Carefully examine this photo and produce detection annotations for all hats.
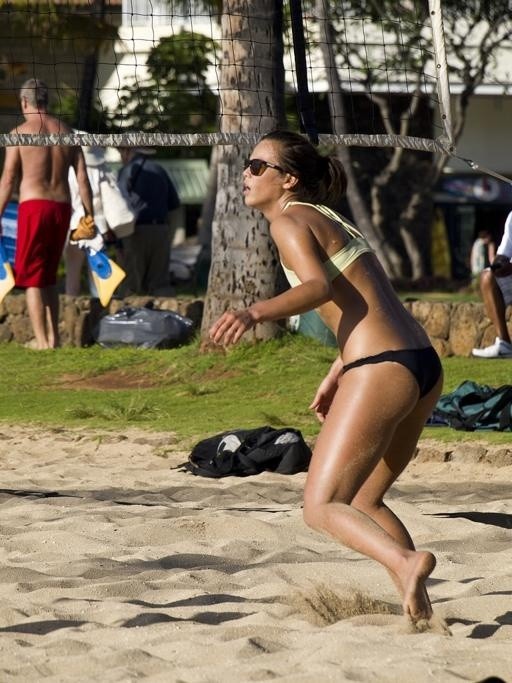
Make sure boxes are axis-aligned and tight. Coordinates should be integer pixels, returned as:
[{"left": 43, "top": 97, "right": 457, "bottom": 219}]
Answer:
[{"left": 72, "top": 130, "right": 105, "bottom": 167}]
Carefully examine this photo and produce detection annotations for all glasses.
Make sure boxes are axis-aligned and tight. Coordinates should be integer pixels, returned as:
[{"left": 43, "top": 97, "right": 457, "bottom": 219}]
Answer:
[{"left": 243, "top": 156, "right": 286, "bottom": 177}]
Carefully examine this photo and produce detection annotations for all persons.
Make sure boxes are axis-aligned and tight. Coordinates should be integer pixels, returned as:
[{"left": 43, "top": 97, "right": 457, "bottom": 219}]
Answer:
[
  {"left": 467, "top": 229, "right": 495, "bottom": 277},
  {"left": 0, "top": 76, "right": 98, "bottom": 352},
  {"left": 115, "top": 129, "right": 183, "bottom": 298},
  {"left": 208, "top": 129, "right": 447, "bottom": 626},
  {"left": 64, "top": 129, "right": 123, "bottom": 295},
  {"left": 469, "top": 207, "right": 511, "bottom": 358}
]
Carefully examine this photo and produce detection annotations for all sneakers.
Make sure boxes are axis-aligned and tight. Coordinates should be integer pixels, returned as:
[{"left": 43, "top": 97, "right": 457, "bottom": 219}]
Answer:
[{"left": 471, "top": 336, "right": 512, "bottom": 360}]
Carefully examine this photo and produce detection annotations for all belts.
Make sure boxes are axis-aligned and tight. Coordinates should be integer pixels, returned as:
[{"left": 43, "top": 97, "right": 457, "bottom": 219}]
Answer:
[{"left": 135, "top": 219, "right": 166, "bottom": 225}]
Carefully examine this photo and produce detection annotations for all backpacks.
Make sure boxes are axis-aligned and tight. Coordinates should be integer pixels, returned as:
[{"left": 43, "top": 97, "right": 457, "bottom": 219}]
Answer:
[
  {"left": 429, "top": 379, "right": 512, "bottom": 434},
  {"left": 172, "top": 425, "right": 312, "bottom": 479}
]
